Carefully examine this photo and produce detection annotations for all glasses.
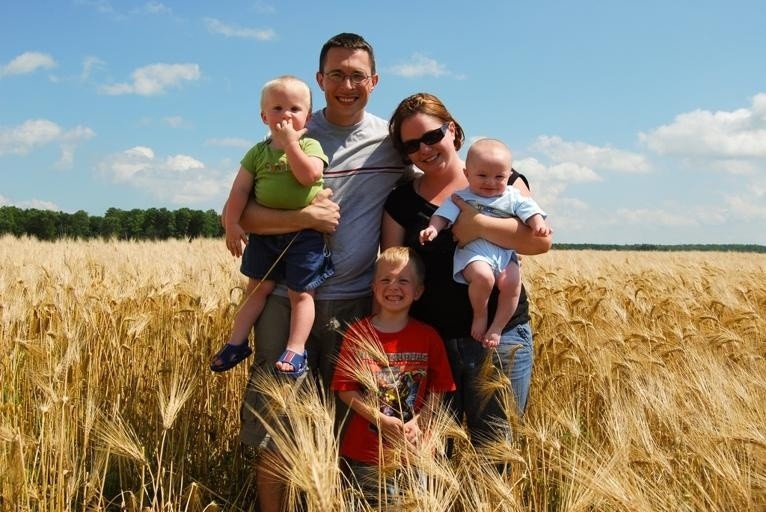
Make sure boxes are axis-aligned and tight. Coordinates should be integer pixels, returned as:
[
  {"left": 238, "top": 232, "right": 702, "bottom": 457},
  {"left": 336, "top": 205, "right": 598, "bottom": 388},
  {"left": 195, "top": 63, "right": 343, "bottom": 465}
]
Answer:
[
  {"left": 402, "top": 123, "right": 449, "bottom": 154},
  {"left": 323, "top": 70, "right": 373, "bottom": 83}
]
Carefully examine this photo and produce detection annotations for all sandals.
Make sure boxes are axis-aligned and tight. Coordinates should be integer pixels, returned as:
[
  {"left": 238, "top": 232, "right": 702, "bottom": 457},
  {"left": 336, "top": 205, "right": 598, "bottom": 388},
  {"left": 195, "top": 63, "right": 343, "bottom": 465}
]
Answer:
[
  {"left": 210, "top": 338, "right": 252, "bottom": 372},
  {"left": 274, "top": 349, "right": 307, "bottom": 376}
]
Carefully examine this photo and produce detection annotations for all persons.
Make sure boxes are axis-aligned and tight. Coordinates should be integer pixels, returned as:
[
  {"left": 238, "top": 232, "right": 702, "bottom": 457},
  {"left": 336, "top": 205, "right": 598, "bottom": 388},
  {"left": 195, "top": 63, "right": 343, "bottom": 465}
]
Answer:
[
  {"left": 240, "top": 32, "right": 414, "bottom": 510},
  {"left": 326, "top": 245, "right": 463, "bottom": 511},
  {"left": 203, "top": 74, "right": 330, "bottom": 375},
  {"left": 419, "top": 141, "right": 552, "bottom": 349},
  {"left": 378, "top": 93, "right": 553, "bottom": 477}
]
